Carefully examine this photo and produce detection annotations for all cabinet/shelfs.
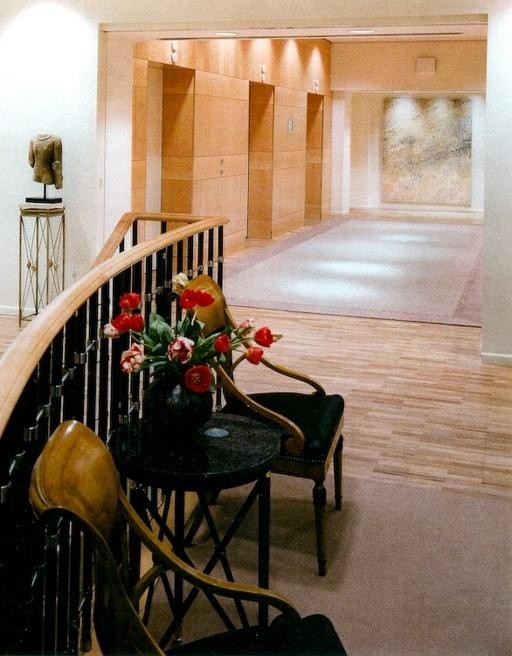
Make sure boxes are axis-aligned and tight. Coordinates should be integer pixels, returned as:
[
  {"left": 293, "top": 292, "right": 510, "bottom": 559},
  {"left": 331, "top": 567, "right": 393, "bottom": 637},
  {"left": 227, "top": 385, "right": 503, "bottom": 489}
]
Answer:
[{"left": 18, "top": 208, "right": 67, "bottom": 327}]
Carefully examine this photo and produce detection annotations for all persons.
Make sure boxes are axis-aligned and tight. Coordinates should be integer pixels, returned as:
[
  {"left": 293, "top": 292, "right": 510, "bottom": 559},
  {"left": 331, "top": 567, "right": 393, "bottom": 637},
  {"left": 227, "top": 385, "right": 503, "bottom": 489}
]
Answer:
[{"left": 29, "top": 133, "right": 62, "bottom": 190}]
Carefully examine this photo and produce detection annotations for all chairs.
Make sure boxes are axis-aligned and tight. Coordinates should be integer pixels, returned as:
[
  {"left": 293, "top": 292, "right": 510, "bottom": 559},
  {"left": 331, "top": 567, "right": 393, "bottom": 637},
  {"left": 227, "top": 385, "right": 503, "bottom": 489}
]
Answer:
[
  {"left": 28, "top": 419, "right": 351, "bottom": 656},
  {"left": 180, "top": 275, "right": 345, "bottom": 578}
]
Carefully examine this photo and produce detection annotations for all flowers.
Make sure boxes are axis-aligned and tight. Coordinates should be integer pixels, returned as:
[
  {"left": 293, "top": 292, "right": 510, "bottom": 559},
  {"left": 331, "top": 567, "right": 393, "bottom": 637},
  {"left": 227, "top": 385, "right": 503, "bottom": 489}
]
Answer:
[{"left": 102, "top": 272, "right": 284, "bottom": 395}]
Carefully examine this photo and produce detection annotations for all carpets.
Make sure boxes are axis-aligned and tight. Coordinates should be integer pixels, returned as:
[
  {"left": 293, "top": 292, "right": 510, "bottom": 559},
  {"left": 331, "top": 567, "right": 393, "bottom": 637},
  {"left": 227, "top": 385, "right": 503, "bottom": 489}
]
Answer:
[{"left": 192, "top": 213, "right": 482, "bottom": 328}]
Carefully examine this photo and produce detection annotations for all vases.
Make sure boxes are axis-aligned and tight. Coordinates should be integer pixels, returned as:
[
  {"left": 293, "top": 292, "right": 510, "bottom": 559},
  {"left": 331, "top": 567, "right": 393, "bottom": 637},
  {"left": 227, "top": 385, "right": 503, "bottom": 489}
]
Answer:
[{"left": 141, "top": 360, "right": 215, "bottom": 437}]
